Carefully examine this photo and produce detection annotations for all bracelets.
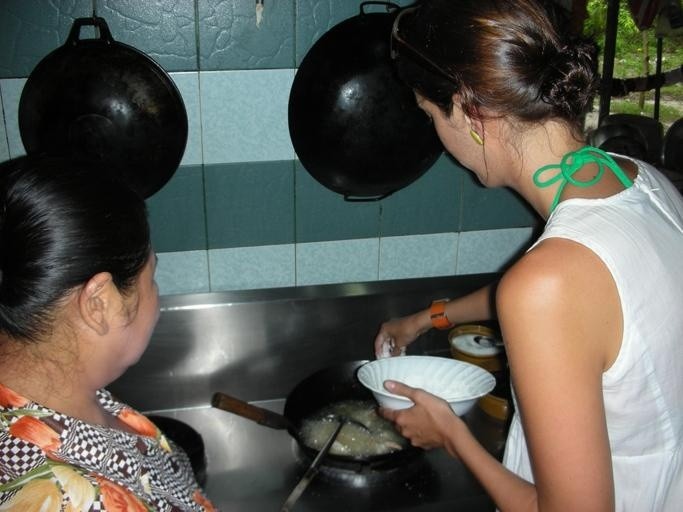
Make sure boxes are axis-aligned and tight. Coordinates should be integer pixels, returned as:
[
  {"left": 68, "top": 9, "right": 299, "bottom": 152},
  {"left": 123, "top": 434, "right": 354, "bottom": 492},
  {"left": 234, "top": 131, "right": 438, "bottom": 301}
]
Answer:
[{"left": 430, "top": 298, "right": 454, "bottom": 331}]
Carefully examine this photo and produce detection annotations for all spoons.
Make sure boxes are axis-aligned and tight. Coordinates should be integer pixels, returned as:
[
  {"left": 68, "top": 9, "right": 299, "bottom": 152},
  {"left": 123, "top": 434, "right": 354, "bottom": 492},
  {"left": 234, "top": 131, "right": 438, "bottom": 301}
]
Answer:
[{"left": 473, "top": 334, "right": 506, "bottom": 349}]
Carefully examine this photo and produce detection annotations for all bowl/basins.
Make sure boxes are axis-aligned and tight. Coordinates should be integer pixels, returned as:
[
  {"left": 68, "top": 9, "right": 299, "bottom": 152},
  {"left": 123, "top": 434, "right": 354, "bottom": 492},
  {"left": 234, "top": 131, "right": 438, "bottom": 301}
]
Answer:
[
  {"left": 479, "top": 387, "right": 514, "bottom": 421},
  {"left": 448, "top": 325, "right": 505, "bottom": 371},
  {"left": 357, "top": 354, "right": 498, "bottom": 421}
]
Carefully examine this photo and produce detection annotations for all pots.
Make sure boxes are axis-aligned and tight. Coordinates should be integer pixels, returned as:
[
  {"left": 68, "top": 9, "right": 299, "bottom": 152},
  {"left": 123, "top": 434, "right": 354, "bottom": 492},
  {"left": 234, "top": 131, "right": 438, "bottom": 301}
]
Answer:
[
  {"left": 207, "top": 361, "right": 426, "bottom": 474},
  {"left": 287, "top": 1, "right": 446, "bottom": 201},
  {"left": 16, "top": 13, "right": 192, "bottom": 204}
]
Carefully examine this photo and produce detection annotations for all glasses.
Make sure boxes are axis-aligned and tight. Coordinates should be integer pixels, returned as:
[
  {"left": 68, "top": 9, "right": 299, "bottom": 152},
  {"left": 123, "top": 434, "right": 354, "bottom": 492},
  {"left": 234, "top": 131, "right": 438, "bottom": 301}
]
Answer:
[{"left": 388, "top": 4, "right": 462, "bottom": 89}]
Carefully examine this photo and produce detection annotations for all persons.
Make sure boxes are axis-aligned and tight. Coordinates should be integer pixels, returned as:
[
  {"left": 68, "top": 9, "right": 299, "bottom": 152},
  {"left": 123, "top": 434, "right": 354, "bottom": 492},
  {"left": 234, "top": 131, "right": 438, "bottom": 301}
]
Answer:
[
  {"left": 0, "top": 145, "right": 218, "bottom": 512},
  {"left": 374, "top": 2, "right": 683, "bottom": 512}
]
